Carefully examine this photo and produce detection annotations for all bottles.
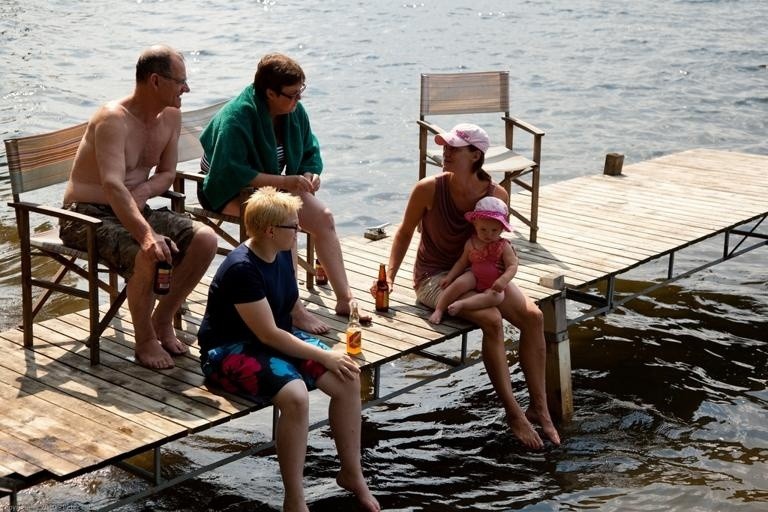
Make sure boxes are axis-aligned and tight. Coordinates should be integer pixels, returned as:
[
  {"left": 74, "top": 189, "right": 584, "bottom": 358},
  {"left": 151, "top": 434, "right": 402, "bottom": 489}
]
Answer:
[
  {"left": 376, "top": 264, "right": 389, "bottom": 313},
  {"left": 155, "top": 238, "right": 176, "bottom": 295},
  {"left": 345, "top": 298, "right": 362, "bottom": 354},
  {"left": 315, "top": 257, "right": 327, "bottom": 285}
]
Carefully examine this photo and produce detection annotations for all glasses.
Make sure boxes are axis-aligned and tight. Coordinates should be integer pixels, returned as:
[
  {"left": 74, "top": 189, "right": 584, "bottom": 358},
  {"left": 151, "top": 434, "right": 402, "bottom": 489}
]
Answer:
[
  {"left": 271, "top": 223, "right": 298, "bottom": 233},
  {"left": 156, "top": 73, "right": 187, "bottom": 85},
  {"left": 280, "top": 82, "right": 306, "bottom": 100}
]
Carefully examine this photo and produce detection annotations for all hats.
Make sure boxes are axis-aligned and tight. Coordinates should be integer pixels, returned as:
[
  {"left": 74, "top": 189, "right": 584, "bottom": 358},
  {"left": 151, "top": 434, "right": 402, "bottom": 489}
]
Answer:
[
  {"left": 435, "top": 123, "right": 490, "bottom": 154},
  {"left": 464, "top": 196, "right": 512, "bottom": 232}
]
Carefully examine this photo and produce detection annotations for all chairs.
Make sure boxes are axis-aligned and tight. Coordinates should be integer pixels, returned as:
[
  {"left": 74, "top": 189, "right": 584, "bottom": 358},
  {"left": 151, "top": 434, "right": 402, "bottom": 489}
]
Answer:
[
  {"left": 0, "top": 119, "right": 187, "bottom": 369},
  {"left": 169, "top": 101, "right": 319, "bottom": 292},
  {"left": 415, "top": 67, "right": 548, "bottom": 245}
]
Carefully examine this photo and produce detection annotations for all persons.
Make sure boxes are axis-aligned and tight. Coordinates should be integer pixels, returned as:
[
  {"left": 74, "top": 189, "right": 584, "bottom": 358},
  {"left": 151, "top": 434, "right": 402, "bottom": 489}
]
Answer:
[
  {"left": 377, "top": 122, "right": 564, "bottom": 452},
  {"left": 198, "top": 54, "right": 372, "bottom": 334},
  {"left": 427, "top": 195, "right": 520, "bottom": 326},
  {"left": 58, "top": 48, "right": 220, "bottom": 371},
  {"left": 199, "top": 186, "right": 385, "bottom": 511}
]
